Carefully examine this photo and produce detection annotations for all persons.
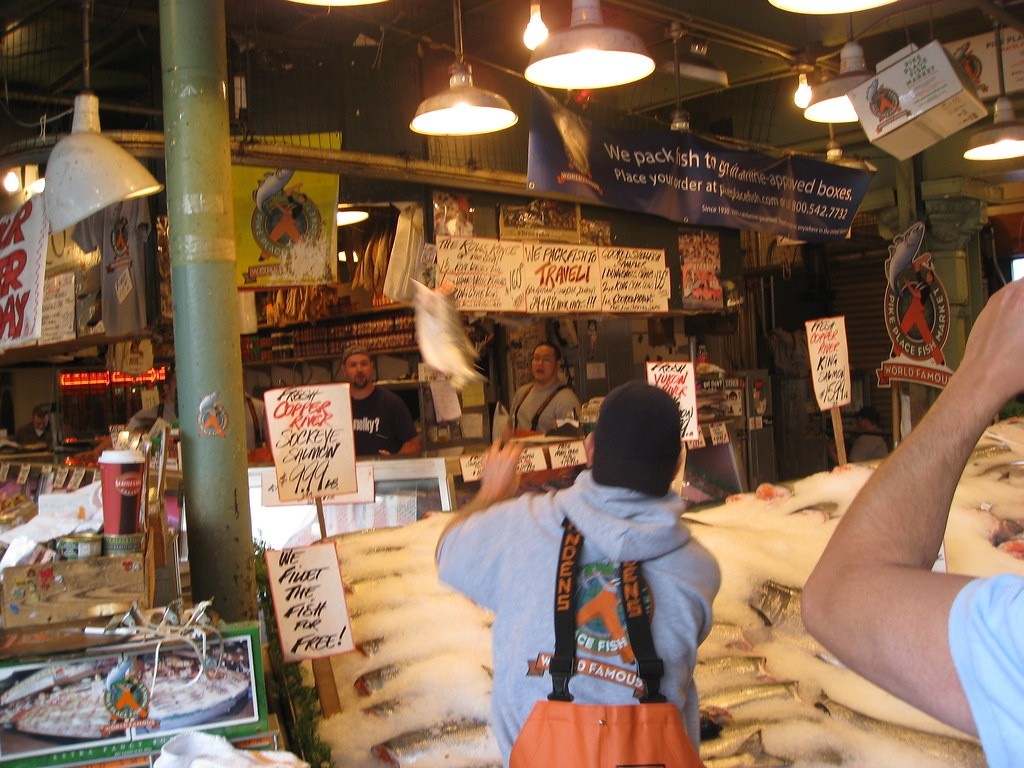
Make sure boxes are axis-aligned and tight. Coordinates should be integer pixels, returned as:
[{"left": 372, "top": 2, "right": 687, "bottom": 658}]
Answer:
[
  {"left": 341, "top": 345, "right": 421, "bottom": 456},
  {"left": 242, "top": 368, "right": 270, "bottom": 451},
  {"left": 435, "top": 381, "right": 723, "bottom": 768},
  {"left": 801, "top": 278, "right": 1024, "bottom": 768},
  {"left": 15, "top": 404, "right": 53, "bottom": 444},
  {"left": 510, "top": 341, "right": 581, "bottom": 435}
]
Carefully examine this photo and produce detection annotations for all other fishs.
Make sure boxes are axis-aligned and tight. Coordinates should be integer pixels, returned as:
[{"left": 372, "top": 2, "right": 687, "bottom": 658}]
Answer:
[
  {"left": 309, "top": 423, "right": 1024, "bottom": 766},
  {"left": 407, "top": 278, "right": 490, "bottom": 391}
]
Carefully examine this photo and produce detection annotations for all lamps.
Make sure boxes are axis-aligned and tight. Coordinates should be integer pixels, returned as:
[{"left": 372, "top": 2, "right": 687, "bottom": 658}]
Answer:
[
  {"left": 409, "top": 0, "right": 518, "bottom": 135},
  {"left": 43, "top": 0, "right": 163, "bottom": 233},
  {"left": 959, "top": 18, "right": 1024, "bottom": 160},
  {"left": 804, "top": 13, "right": 879, "bottom": 124},
  {"left": 526, "top": 0, "right": 656, "bottom": 93}
]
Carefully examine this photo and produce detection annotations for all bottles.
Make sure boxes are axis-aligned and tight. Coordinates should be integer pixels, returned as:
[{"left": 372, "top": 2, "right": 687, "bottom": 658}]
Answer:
[{"left": 102, "top": 534, "right": 145, "bottom": 555}]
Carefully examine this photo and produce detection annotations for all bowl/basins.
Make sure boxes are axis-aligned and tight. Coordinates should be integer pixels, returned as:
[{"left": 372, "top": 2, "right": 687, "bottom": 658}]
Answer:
[{"left": 57, "top": 532, "right": 103, "bottom": 561}]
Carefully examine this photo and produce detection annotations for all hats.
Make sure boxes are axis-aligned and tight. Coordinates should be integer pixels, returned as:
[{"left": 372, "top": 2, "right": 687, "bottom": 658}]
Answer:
[
  {"left": 342, "top": 346, "right": 371, "bottom": 366},
  {"left": 592, "top": 381, "right": 682, "bottom": 497}
]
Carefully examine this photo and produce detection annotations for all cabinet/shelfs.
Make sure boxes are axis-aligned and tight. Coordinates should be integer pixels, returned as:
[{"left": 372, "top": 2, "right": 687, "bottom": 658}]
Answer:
[{"left": 240, "top": 304, "right": 420, "bottom": 368}]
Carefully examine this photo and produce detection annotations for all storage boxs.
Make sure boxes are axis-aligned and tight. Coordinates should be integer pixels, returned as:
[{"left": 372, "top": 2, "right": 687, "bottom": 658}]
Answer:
[
  {"left": 846, "top": 28, "right": 1024, "bottom": 162},
  {"left": 0, "top": 526, "right": 163, "bottom": 659}
]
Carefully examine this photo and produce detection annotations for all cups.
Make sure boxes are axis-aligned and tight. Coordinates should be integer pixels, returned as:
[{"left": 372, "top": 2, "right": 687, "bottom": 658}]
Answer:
[{"left": 98, "top": 450, "right": 146, "bottom": 534}]
